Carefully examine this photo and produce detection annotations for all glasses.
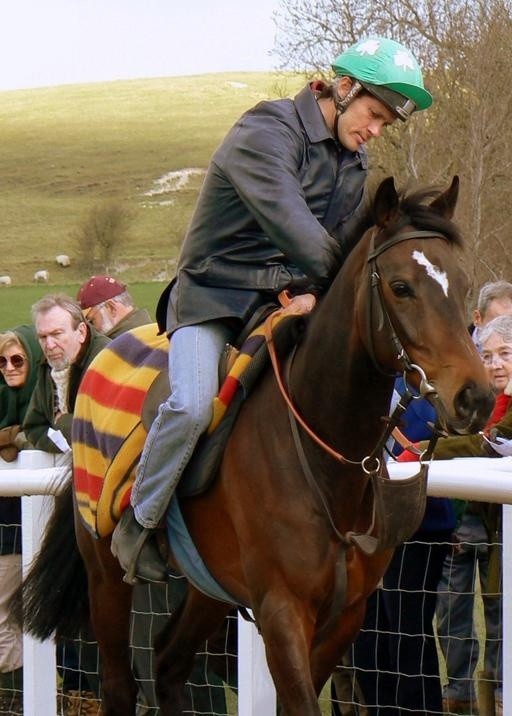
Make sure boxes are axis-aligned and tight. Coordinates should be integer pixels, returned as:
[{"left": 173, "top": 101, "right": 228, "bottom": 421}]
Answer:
[
  {"left": 88, "top": 304, "right": 105, "bottom": 325},
  {"left": 0, "top": 353, "right": 26, "bottom": 369}
]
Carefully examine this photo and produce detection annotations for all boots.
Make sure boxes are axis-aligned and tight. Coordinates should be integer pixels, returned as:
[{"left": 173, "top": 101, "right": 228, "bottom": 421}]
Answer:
[{"left": 110, "top": 506, "right": 170, "bottom": 585}]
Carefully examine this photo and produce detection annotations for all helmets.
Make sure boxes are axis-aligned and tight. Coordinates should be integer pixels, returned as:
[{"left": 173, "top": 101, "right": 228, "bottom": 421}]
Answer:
[{"left": 330, "top": 36, "right": 433, "bottom": 123}]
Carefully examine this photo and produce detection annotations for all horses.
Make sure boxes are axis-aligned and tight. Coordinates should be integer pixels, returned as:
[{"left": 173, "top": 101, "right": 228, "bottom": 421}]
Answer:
[{"left": 0, "top": 171, "right": 499, "bottom": 716}]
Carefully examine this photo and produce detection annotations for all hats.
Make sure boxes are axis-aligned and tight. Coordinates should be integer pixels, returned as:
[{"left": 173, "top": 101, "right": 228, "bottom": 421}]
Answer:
[{"left": 77, "top": 275, "right": 126, "bottom": 317}]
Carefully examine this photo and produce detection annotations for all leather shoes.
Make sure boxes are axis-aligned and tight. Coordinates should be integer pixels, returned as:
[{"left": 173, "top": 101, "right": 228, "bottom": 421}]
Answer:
[{"left": 442, "top": 695, "right": 478, "bottom": 714}]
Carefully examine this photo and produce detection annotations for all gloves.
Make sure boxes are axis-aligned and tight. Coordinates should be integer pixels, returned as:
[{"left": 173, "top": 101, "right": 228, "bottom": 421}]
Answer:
[
  {"left": 0, "top": 445, "right": 18, "bottom": 463},
  {"left": 0, "top": 424, "right": 22, "bottom": 450}
]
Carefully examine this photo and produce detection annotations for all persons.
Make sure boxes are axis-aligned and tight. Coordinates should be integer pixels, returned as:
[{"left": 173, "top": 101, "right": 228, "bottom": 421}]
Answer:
[
  {"left": 110, "top": 34, "right": 436, "bottom": 585},
  {"left": 0, "top": 276, "right": 512, "bottom": 716}
]
